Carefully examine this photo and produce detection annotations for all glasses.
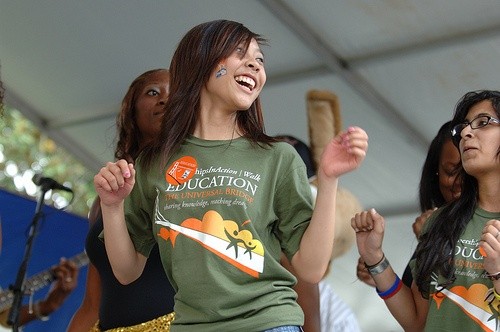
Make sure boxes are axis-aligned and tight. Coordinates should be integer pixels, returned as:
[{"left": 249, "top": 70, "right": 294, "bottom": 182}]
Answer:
[{"left": 451, "top": 115, "right": 500, "bottom": 137}]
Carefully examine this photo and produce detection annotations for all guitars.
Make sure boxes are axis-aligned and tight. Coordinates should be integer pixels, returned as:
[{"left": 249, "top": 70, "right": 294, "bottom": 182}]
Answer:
[{"left": 0, "top": 247, "right": 91, "bottom": 314}]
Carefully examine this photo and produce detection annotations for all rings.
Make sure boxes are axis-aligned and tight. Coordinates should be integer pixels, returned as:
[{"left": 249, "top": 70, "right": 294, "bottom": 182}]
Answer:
[
  {"left": 66, "top": 277, "right": 72, "bottom": 282},
  {"left": 496, "top": 231, "right": 500, "bottom": 238}
]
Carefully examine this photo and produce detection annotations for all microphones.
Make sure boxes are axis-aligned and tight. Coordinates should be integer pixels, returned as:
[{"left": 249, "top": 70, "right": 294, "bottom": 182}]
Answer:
[{"left": 32, "top": 173, "right": 72, "bottom": 192}]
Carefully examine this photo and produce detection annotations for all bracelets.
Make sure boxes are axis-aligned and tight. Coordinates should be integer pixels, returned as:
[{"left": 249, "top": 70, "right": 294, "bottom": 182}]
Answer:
[
  {"left": 487, "top": 272, "right": 500, "bottom": 281},
  {"left": 35, "top": 301, "right": 49, "bottom": 321},
  {"left": 376, "top": 275, "right": 400, "bottom": 296},
  {"left": 364, "top": 254, "right": 389, "bottom": 274},
  {"left": 380, "top": 280, "right": 402, "bottom": 300},
  {"left": 493, "top": 287, "right": 500, "bottom": 300}
]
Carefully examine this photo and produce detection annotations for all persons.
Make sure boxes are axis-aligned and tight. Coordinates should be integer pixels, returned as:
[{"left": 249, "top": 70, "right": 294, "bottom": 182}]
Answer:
[
  {"left": 0, "top": 257, "right": 77, "bottom": 332},
  {"left": 351, "top": 89, "right": 500, "bottom": 332},
  {"left": 356, "top": 120, "right": 462, "bottom": 288},
  {"left": 67, "top": 69, "right": 320, "bottom": 332},
  {"left": 95, "top": 20, "right": 368, "bottom": 332}
]
